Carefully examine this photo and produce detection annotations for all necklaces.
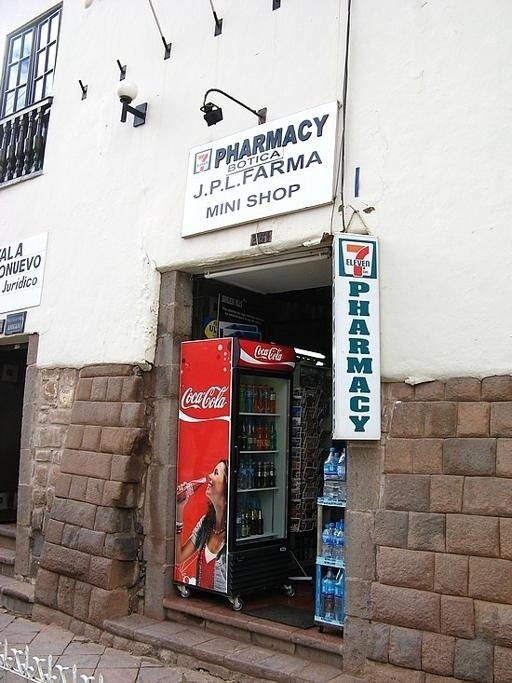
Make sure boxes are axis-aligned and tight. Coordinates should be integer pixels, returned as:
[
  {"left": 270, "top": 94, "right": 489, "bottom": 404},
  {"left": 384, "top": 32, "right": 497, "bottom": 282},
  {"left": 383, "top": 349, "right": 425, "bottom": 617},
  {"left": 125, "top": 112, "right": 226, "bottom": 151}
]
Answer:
[{"left": 211, "top": 526, "right": 226, "bottom": 537}]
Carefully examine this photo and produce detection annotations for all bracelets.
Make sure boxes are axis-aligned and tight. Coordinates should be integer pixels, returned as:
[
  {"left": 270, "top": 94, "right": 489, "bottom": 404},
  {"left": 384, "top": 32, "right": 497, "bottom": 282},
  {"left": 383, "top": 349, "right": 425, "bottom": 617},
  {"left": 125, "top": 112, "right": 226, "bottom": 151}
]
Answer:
[{"left": 176, "top": 521, "right": 183, "bottom": 537}]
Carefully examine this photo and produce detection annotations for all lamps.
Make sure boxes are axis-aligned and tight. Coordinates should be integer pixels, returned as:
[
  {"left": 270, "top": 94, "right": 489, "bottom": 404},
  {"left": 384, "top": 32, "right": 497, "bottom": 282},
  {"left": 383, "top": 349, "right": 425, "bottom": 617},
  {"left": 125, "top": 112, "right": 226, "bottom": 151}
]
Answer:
[
  {"left": 199, "top": 89, "right": 267, "bottom": 126},
  {"left": 116, "top": 83, "right": 148, "bottom": 127}
]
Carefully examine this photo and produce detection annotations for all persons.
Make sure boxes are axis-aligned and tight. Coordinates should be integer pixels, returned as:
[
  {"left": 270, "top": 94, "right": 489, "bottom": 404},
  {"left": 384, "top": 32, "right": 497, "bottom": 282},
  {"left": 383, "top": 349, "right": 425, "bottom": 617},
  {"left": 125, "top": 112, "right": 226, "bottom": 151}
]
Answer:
[{"left": 176, "top": 459, "right": 228, "bottom": 593}]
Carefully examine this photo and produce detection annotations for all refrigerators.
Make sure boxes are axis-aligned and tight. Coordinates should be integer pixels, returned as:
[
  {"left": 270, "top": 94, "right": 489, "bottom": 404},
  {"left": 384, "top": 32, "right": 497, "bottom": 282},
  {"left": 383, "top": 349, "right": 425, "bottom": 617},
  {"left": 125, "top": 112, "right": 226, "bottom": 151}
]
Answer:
[{"left": 174, "top": 335, "right": 297, "bottom": 602}]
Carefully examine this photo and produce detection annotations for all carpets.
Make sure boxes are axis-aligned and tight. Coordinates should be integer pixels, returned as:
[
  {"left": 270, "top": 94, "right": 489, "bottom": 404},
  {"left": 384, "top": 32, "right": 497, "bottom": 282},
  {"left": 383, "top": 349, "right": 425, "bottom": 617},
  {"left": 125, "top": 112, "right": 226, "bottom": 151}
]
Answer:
[{"left": 241, "top": 603, "right": 318, "bottom": 631}]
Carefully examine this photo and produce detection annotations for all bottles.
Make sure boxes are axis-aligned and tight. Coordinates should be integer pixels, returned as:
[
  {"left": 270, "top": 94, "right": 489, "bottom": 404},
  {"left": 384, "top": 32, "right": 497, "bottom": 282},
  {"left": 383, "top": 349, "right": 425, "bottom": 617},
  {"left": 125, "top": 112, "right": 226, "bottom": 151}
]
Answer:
[
  {"left": 322, "top": 447, "right": 345, "bottom": 625},
  {"left": 238, "top": 382, "right": 277, "bottom": 538}
]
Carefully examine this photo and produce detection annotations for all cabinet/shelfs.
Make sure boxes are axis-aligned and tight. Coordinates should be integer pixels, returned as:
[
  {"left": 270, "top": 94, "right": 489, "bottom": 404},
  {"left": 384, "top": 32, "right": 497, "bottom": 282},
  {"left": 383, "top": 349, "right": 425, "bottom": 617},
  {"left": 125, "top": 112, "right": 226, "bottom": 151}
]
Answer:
[{"left": 313, "top": 497, "right": 347, "bottom": 633}]
